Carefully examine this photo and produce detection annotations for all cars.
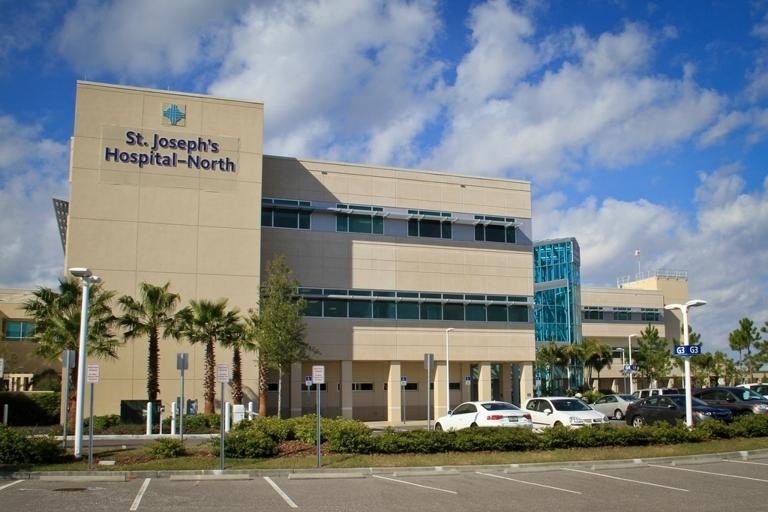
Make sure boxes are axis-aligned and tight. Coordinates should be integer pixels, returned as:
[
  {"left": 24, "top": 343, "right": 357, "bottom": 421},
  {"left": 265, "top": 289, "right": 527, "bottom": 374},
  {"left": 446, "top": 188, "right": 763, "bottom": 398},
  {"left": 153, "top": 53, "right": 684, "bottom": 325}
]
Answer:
[
  {"left": 523, "top": 396, "right": 611, "bottom": 432},
  {"left": 433, "top": 400, "right": 534, "bottom": 432},
  {"left": 591, "top": 381, "right": 768, "bottom": 431}
]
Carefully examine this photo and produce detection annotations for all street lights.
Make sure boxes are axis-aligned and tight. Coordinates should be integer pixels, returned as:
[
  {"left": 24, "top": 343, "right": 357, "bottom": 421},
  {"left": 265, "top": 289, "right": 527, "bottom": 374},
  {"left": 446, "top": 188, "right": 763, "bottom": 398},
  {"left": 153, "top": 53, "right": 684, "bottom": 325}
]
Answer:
[
  {"left": 663, "top": 300, "right": 707, "bottom": 431},
  {"left": 616, "top": 347, "right": 627, "bottom": 394},
  {"left": 445, "top": 327, "right": 455, "bottom": 414},
  {"left": 68, "top": 266, "right": 102, "bottom": 460},
  {"left": 628, "top": 333, "right": 637, "bottom": 365}
]
